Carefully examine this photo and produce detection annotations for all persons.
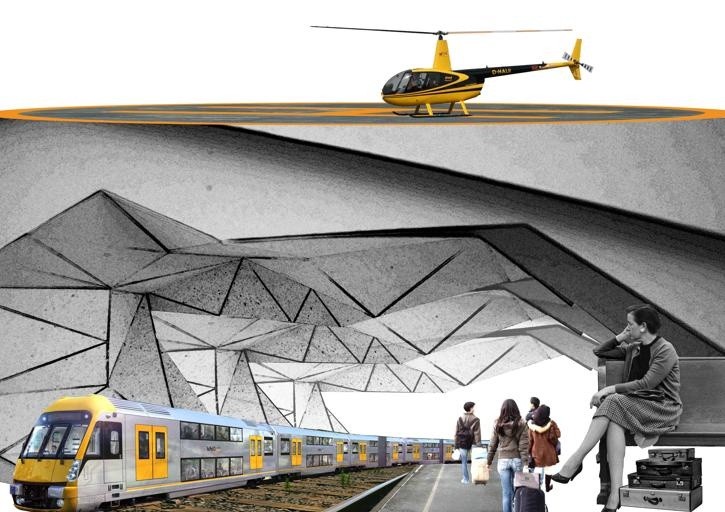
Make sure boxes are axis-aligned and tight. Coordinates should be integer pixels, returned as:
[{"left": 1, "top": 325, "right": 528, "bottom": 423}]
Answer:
[
  {"left": 526, "top": 403, "right": 558, "bottom": 491},
  {"left": 485, "top": 397, "right": 531, "bottom": 512},
  {"left": 179, "top": 424, "right": 241, "bottom": 480},
  {"left": 525, "top": 396, "right": 540, "bottom": 425},
  {"left": 553, "top": 302, "right": 684, "bottom": 512},
  {"left": 455, "top": 402, "right": 484, "bottom": 484}
]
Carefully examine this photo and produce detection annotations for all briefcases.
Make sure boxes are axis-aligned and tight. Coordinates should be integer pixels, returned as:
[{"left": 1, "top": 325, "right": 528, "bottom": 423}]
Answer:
[{"left": 618, "top": 448, "right": 704, "bottom": 511}]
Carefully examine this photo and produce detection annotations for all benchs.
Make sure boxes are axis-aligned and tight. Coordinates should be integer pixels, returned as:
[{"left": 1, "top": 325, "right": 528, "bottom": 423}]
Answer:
[{"left": 596, "top": 357, "right": 725, "bottom": 506}]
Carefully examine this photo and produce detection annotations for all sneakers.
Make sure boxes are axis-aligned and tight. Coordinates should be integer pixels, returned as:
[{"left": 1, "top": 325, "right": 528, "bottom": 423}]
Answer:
[
  {"left": 546, "top": 484, "right": 553, "bottom": 492},
  {"left": 461, "top": 478, "right": 470, "bottom": 485}
]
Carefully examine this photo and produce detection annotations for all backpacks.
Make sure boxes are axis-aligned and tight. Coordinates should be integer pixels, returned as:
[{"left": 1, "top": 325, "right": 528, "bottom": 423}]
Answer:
[{"left": 455, "top": 417, "right": 478, "bottom": 450}]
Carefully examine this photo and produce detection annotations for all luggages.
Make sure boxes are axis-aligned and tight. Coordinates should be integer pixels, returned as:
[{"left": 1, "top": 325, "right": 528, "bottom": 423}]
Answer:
[
  {"left": 471, "top": 444, "right": 490, "bottom": 486},
  {"left": 511, "top": 457, "right": 548, "bottom": 512}
]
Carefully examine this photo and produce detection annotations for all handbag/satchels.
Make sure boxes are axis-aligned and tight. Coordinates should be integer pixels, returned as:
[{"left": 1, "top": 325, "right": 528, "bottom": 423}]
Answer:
[
  {"left": 546, "top": 426, "right": 558, "bottom": 447},
  {"left": 626, "top": 388, "right": 666, "bottom": 402},
  {"left": 513, "top": 468, "right": 541, "bottom": 492},
  {"left": 472, "top": 447, "right": 487, "bottom": 459},
  {"left": 452, "top": 448, "right": 461, "bottom": 461}
]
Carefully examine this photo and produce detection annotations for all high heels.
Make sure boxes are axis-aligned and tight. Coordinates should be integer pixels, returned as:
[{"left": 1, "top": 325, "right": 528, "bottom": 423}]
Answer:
[
  {"left": 601, "top": 489, "right": 622, "bottom": 512},
  {"left": 551, "top": 462, "right": 582, "bottom": 484}
]
